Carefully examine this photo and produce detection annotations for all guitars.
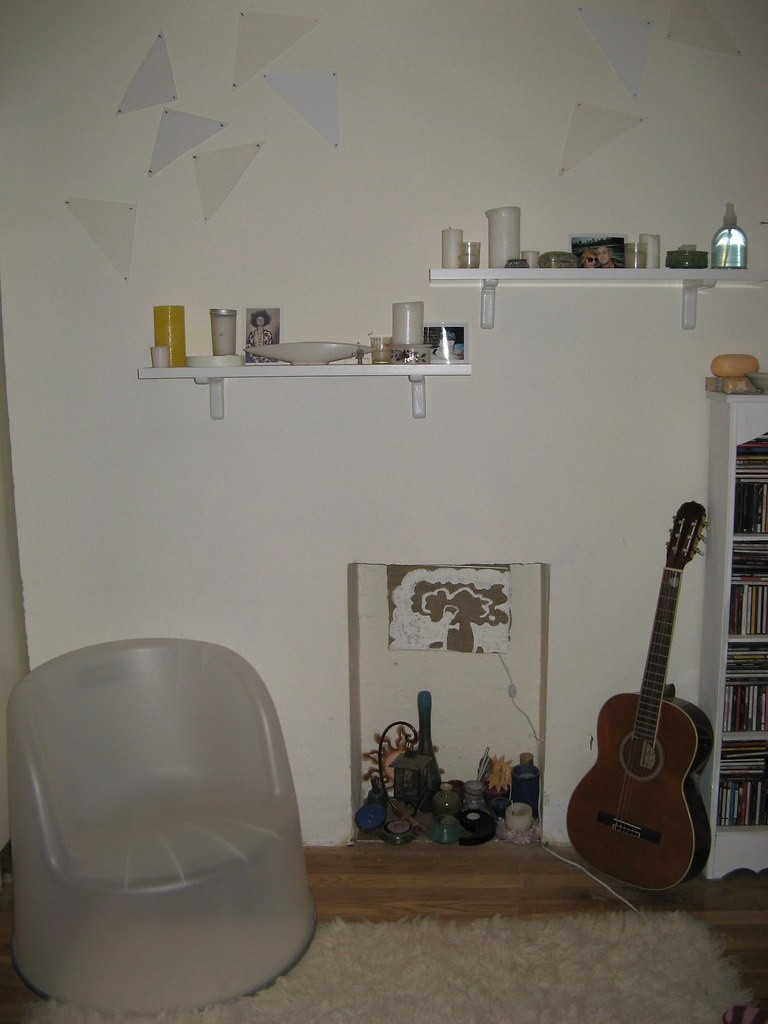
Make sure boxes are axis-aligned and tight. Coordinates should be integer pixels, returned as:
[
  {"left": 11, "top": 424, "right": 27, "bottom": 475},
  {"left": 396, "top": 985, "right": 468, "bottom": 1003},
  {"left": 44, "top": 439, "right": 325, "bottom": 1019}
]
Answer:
[{"left": 567, "top": 493, "right": 717, "bottom": 895}]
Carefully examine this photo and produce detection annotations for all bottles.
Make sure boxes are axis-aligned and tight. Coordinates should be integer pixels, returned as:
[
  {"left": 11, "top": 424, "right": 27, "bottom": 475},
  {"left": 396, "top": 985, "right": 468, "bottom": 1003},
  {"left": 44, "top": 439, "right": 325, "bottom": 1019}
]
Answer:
[
  {"left": 511, "top": 752, "right": 539, "bottom": 820},
  {"left": 368, "top": 778, "right": 387, "bottom": 809},
  {"left": 432, "top": 783, "right": 461, "bottom": 816}
]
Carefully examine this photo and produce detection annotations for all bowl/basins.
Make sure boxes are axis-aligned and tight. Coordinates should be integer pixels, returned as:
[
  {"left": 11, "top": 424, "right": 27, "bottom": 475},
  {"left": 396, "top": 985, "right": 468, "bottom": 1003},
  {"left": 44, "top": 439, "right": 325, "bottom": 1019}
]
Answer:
[{"left": 747, "top": 372, "right": 768, "bottom": 394}]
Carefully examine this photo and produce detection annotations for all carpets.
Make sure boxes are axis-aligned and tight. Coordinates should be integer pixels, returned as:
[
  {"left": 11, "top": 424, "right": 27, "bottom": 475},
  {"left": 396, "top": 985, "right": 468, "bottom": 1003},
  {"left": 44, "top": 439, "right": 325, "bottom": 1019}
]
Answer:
[{"left": 14, "top": 912, "right": 754, "bottom": 1024}]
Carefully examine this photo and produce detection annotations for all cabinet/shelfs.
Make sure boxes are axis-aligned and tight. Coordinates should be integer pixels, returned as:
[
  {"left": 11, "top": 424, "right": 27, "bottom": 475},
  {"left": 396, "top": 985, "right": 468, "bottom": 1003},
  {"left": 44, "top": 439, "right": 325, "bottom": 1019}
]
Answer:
[
  {"left": 698, "top": 388, "right": 768, "bottom": 885},
  {"left": 137, "top": 265, "right": 766, "bottom": 418}
]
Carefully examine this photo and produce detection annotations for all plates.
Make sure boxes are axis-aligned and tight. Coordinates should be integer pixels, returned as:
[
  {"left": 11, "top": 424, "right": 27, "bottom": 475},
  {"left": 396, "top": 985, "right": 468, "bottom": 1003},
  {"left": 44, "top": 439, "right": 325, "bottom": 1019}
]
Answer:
[{"left": 242, "top": 343, "right": 371, "bottom": 367}]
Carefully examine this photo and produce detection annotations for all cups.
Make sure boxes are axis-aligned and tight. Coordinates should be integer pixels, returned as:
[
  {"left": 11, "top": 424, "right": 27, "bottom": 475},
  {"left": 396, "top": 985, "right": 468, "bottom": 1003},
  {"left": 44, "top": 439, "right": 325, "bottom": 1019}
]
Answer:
[
  {"left": 460, "top": 241, "right": 482, "bottom": 268},
  {"left": 626, "top": 242, "right": 647, "bottom": 269},
  {"left": 151, "top": 345, "right": 170, "bottom": 368},
  {"left": 522, "top": 250, "right": 540, "bottom": 268},
  {"left": 368, "top": 337, "right": 394, "bottom": 364},
  {"left": 505, "top": 803, "right": 532, "bottom": 833},
  {"left": 210, "top": 309, "right": 238, "bottom": 356}
]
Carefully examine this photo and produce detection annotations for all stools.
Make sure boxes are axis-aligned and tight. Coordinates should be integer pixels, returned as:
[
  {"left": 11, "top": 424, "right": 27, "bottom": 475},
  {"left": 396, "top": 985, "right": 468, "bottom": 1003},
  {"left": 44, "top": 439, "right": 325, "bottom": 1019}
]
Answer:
[{"left": 5, "top": 639, "right": 321, "bottom": 1008}]
